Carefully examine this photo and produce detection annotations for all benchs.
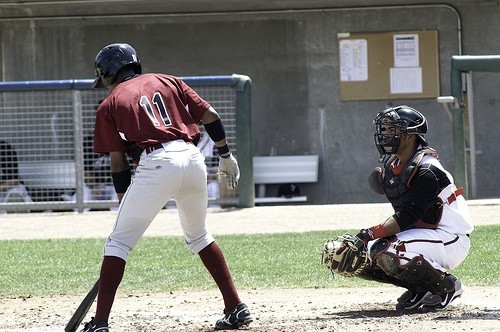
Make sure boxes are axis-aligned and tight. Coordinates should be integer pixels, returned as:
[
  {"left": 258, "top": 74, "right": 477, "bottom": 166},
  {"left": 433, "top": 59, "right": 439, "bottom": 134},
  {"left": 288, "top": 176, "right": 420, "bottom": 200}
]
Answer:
[{"left": 18, "top": 155, "right": 319, "bottom": 202}]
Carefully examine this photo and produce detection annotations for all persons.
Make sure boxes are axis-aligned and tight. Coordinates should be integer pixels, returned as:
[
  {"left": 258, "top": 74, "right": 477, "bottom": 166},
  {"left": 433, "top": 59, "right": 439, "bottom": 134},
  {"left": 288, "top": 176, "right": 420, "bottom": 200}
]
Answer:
[
  {"left": 0, "top": 99, "right": 283, "bottom": 214},
  {"left": 323, "top": 105, "right": 475, "bottom": 311},
  {"left": 77, "top": 43, "right": 253, "bottom": 332}
]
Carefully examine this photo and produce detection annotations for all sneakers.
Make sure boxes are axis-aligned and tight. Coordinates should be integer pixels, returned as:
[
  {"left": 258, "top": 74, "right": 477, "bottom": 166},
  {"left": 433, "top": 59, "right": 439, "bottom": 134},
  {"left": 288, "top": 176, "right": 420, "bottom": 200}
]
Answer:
[
  {"left": 215, "top": 302, "right": 253, "bottom": 330},
  {"left": 79, "top": 317, "right": 111, "bottom": 332},
  {"left": 418, "top": 274, "right": 464, "bottom": 313},
  {"left": 396, "top": 287, "right": 433, "bottom": 311}
]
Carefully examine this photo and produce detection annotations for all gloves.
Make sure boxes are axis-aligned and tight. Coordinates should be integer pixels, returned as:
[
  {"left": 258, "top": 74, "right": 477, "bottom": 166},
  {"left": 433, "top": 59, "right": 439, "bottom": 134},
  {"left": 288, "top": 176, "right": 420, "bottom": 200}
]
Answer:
[{"left": 217, "top": 151, "right": 240, "bottom": 189}]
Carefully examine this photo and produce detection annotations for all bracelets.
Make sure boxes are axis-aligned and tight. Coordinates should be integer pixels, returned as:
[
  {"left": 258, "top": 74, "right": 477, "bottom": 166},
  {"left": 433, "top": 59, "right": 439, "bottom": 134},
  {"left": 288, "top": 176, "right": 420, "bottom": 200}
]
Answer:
[
  {"left": 369, "top": 224, "right": 387, "bottom": 238},
  {"left": 218, "top": 143, "right": 230, "bottom": 155}
]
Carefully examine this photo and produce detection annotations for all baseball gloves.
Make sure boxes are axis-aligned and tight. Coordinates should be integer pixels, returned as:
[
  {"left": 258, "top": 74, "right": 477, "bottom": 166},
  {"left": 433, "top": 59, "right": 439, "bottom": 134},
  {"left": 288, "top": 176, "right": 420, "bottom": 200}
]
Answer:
[{"left": 321, "top": 232, "right": 368, "bottom": 277}]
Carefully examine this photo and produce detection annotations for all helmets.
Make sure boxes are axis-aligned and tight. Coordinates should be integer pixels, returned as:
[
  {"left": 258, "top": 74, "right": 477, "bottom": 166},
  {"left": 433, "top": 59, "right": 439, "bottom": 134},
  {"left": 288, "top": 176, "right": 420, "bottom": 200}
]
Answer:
[
  {"left": 92, "top": 43, "right": 142, "bottom": 89},
  {"left": 381, "top": 105, "right": 429, "bottom": 146}
]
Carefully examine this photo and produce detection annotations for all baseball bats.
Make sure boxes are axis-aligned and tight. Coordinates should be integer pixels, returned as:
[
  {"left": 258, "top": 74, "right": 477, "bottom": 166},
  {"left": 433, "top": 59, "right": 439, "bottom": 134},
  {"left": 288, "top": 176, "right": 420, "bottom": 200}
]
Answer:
[{"left": 64, "top": 277, "right": 101, "bottom": 332}]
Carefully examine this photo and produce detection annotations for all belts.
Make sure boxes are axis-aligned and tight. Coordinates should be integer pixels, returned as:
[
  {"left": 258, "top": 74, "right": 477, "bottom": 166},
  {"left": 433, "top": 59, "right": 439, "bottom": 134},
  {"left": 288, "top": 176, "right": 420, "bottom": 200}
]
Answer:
[{"left": 146, "top": 139, "right": 190, "bottom": 154}]
